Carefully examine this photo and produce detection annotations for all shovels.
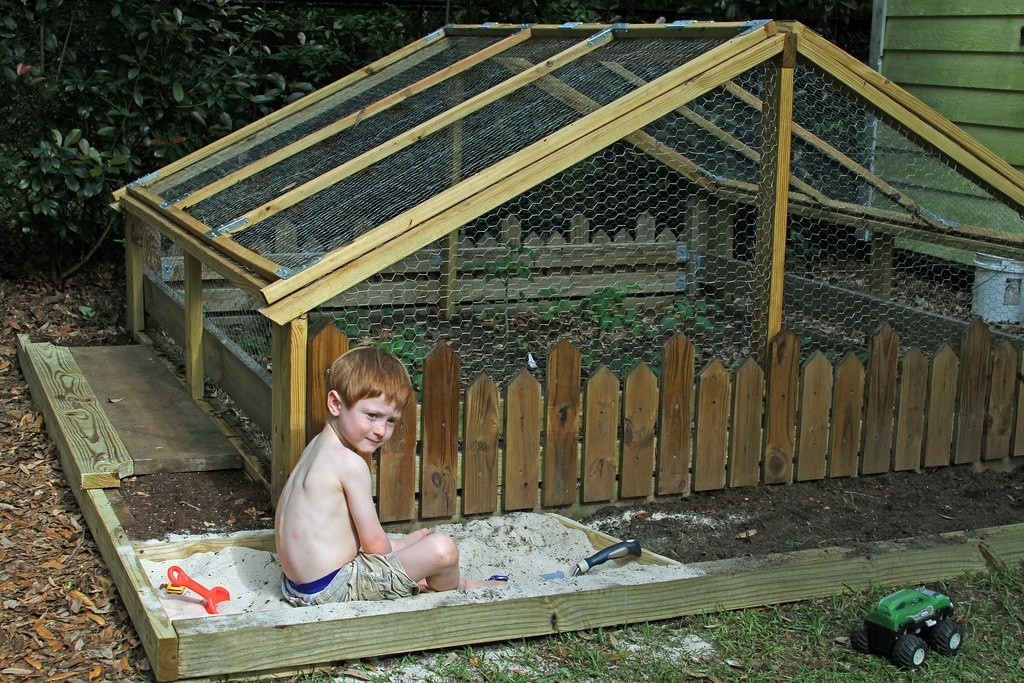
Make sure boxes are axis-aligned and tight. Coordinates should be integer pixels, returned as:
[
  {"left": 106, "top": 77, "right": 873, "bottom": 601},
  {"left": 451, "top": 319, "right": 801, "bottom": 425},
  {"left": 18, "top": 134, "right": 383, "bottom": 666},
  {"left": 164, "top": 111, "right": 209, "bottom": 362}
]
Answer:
[{"left": 166, "top": 564, "right": 231, "bottom": 614}]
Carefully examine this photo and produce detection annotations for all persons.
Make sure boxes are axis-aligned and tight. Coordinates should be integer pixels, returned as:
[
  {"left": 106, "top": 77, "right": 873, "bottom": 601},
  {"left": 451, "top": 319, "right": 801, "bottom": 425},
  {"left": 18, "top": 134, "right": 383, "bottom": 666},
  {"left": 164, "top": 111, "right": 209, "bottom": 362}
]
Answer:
[{"left": 274, "top": 347, "right": 508, "bottom": 608}]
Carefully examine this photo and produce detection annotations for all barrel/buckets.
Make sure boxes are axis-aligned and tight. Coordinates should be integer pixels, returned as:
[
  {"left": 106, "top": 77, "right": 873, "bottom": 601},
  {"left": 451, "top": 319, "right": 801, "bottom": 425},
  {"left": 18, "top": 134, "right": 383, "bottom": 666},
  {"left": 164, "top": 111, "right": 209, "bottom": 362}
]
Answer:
[{"left": 971, "top": 249, "right": 1024, "bottom": 324}]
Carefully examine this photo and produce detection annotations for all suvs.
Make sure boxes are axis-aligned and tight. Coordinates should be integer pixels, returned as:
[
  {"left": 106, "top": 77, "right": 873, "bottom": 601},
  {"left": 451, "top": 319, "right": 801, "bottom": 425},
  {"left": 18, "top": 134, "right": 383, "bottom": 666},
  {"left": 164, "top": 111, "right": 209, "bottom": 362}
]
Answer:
[{"left": 850, "top": 584, "right": 963, "bottom": 672}]
